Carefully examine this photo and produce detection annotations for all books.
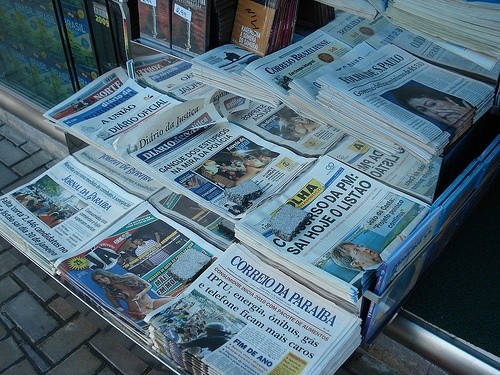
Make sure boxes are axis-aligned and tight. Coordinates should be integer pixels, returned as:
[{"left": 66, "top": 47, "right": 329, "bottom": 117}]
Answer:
[
  {"left": 138, "top": 0, "right": 172, "bottom": 55},
  {"left": 417, "top": 134, "right": 500, "bottom": 287},
  {"left": 172, "top": 0, "right": 210, "bottom": 58},
  {"left": 361, "top": 206, "right": 443, "bottom": 344},
  {"left": 230, "top": 0, "right": 300, "bottom": 59}
]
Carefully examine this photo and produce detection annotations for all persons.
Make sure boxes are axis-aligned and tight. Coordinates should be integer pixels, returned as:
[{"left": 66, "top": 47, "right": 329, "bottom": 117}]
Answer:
[
  {"left": 92, "top": 269, "right": 176, "bottom": 321},
  {"left": 330, "top": 242, "right": 387, "bottom": 286},
  {"left": 71, "top": 103, "right": 81, "bottom": 112},
  {"left": 153, "top": 294, "right": 225, "bottom": 342},
  {"left": 177, "top": 322, "right": 230, "bottom": 353},
  {"left": 395, "top": 86, "right": 473, "bottom": 126},
  {"left": 278, "top": 75, "right": 294, "bottom": 90},
  {"left": 224, "top": 204, "right": 245, "bottom": 216},
  {"left": 77, "top": 98, "right": 93, "bottom": 109},
  {"left": 217, "top": 158, "right": 248, "bottom": 181},
  {"left": 150, "top": 220, "right": 176, "bottom": 244},
  {"left": 117, "top": 247, "right": 154, "bottom": 275},
  {"left": 12, "top": 184, "right": 73, "bottom": 229},
  {"left": 132, "top": 236, "right": 171, "bottom": 269}
]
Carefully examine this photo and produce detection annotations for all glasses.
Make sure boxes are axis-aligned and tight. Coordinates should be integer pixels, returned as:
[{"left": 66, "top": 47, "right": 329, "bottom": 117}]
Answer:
[{"left": 352, "top": 243, "right": 368, "bottom": 260}]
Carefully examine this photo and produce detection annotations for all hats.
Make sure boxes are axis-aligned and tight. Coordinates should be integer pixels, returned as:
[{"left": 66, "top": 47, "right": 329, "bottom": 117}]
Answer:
[{"left": 204, "top": 322, "right": 232, "bottom": 335}]
